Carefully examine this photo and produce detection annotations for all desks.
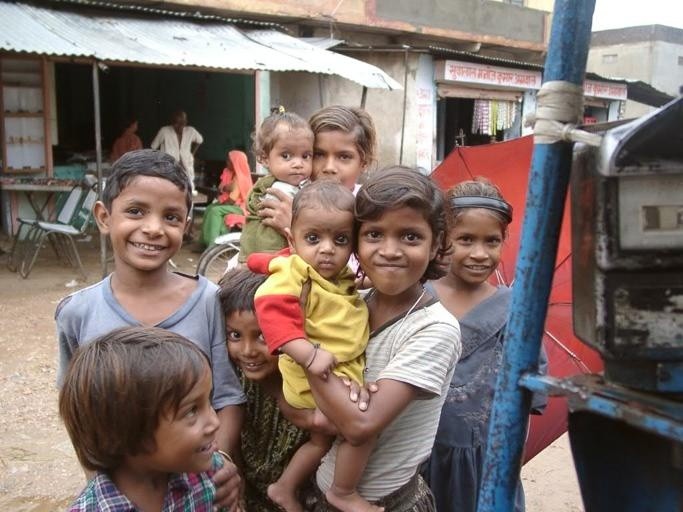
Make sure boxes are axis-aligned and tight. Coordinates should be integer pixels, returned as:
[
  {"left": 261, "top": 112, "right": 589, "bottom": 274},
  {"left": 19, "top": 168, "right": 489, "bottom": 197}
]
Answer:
[{"left": 0, "top": 181, "right": 72, "bottom": 256}]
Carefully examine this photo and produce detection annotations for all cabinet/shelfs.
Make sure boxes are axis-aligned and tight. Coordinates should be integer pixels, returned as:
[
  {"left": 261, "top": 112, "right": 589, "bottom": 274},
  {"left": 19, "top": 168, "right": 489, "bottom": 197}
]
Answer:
[{"left": 0, "top": 54, "right": 54, "bottom": 178}]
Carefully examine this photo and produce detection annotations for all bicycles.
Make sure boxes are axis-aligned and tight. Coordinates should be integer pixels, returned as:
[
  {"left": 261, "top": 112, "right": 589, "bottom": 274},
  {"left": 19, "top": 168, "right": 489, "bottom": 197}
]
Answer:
[{"left": 195, "top": 231, "right": 242, "bottom": 284}]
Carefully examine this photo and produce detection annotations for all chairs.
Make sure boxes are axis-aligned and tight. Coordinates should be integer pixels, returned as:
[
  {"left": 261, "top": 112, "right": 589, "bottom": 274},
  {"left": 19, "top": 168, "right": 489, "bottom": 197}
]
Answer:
[
  {"left": 18, "top": 183, "right": 99, "bottom": 278},
  {"left": 222, "top": 173, "right": 266, "bottom": 232},
  {"left": 6, "top": 186, "right": 83, "bottom": 272}
]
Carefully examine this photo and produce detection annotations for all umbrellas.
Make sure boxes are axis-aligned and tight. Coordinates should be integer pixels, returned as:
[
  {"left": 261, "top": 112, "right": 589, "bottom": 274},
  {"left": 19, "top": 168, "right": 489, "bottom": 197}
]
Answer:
[{"left": 427, "top": 133, "right": 604, "bottom": 470}]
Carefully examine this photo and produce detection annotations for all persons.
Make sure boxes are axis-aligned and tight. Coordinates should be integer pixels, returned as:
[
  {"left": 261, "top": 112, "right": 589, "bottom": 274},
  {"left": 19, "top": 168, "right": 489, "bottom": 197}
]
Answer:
[
  {"left": 215, "top": 103, "right": 546, "bottom": 512},
  {"left": 192, "top": 150, "right": 253, "bottom": 253},
  {"left": 112, "top": 116, "right": 142, "bottom": 165},
  {"left": 151, "top": 110, "right": 203, "bottom": 191},
  {"left": 57, "top": 324, "right": 236, "bottom": 512},
  {"left": 54, "top": 148, "right": 246, "bottom": 512}
]
